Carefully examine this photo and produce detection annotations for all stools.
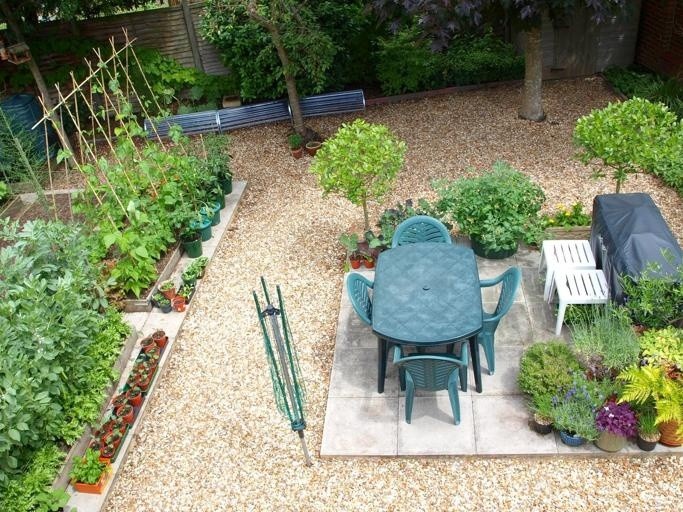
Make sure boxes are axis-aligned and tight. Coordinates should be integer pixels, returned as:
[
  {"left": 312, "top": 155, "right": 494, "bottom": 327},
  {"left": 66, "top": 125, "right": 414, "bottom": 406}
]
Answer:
[
  {"left": 548, "top": 269, "right": 610, "bottom": 336},
  {"left": 538, "top": 240, "right": 596, "bottom": 301}
]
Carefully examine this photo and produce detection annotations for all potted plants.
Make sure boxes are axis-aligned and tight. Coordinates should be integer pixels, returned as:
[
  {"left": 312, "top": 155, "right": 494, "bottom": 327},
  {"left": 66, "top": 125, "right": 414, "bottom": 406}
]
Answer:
[
  {"left": 150, "top": 292, "right": 165, "bottom": 308},
  {"left": 287, "top": 132, "right": 304, "bottom": 159},
  {"left": 158, "top": 299, "right": 171, "bottom": 313},
  {"left": 190, "top": 256, "right": 210, "bottom": 279},
  {"left": 113, "top": 395, "right": 128, "bottom": 408},
  {"left": 126, "top": 390, "right": 141, "bottom": 407},
  {"left": 175, "top": 283, "right": 195, "bottom": 305},
  {"left": 360, "top": 249, "right": 374, "bottom": 268},
  {"left": 171, "top": 296, "right": 186, "bottom": 313},
  {"left": 304, "top": 130, "right": 323, "bottom": 156},
  {"left": 126, "top": 345, "right": 159, "bottom": 391},
  {"left": 157, "top": 276, "right": 176, "bottom": 299},
  {"left": 106, "top": 431, "right": 122, "bottom": 447},
  {"left": 101, "top": 446, "right": 116, "bottom": 457},
  {"left": 638, "top": 409, "right": 661, "bottom": 452},
  {"left": 165, "top": 127, "right": 234, "bottom": 259},
  {"left": 180, "top": 265, "right": 198, "bottom": 287},
  {"left": 336, "top": 230, "right": 373, "bottom": 269},
  {"left": 117, "top": 405, "right": 134, "bottom": 422},
  {"left": 110, "top": 421, "right": 126, "bottom": 432},
  {"left": 70, "top": 447, "right": 111, "bottom": 495},
  {"left": 593, "top": 399, "right": 639, "bottom": 452},
  {"left": 616, "top": 364, "right": 683, "bottom": 447},
  {"left": 524, "top": 393, "right": 556, "bottom": 435},
  {"left": 140, "top": 332, "right": 154, "bottom": 354},
  {"left": 549, "top": 382, "right": 607, "bottom": 445}
]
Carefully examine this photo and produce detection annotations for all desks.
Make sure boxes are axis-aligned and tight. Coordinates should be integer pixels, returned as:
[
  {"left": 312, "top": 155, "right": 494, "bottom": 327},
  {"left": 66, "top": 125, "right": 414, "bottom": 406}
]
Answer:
[{"left": 371, "top": 242, "right": 483, "bottom": 398}]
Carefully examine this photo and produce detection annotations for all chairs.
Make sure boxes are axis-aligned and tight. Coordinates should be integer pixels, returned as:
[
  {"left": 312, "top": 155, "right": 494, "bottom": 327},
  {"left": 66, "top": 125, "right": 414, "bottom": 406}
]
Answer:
[
  {"left": 393, "top": 342, "right": 469, "bottom": 426},
  {"left": 392, "top": 215, "right": 452, "bottom": 247},
  {"left": 430, "top": 265, "right": 521, "bottom": 375},
  {"left": 347, "top": 272, "right": 374, "bottom": 325}
]
